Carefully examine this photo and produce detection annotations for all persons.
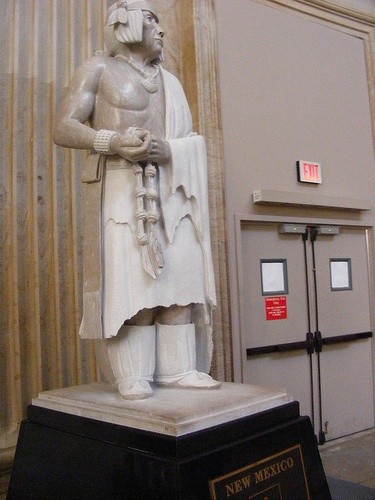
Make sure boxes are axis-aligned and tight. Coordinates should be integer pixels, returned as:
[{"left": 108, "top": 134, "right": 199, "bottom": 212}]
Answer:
[{"left": 51, "top": 0, "right": 221, "bottom": 402}]
